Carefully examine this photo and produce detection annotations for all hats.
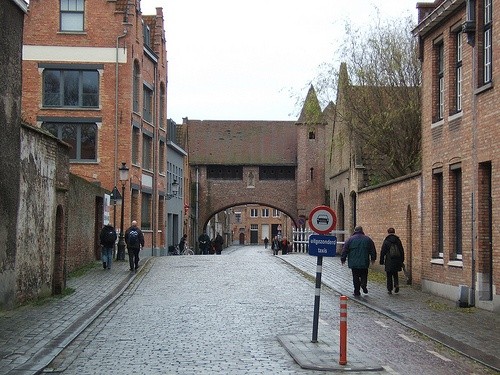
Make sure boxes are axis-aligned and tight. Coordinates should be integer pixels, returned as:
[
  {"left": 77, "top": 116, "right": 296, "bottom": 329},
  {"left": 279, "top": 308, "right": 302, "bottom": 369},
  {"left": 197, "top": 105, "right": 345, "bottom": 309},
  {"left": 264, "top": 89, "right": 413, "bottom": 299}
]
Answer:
[
  {"left": 132, "top": 221, "right": 137, "bottom": 224},
  {"left": 354, "top": 226, "right": 362, "bottom": 232}
]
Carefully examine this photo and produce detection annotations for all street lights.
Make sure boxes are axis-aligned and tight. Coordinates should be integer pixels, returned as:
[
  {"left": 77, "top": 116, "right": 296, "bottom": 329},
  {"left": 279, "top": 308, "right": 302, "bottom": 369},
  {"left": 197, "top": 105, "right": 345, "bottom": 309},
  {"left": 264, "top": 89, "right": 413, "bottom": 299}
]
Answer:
[{"left": 116, "top": 161, "right": 129, "bottom": 261}]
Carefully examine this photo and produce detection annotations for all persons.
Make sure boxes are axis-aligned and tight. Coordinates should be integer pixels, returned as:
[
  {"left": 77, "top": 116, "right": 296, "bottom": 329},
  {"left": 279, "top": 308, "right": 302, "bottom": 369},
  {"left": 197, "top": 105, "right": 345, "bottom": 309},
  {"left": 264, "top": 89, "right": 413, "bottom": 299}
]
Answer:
[
  {"left": 272, "top": 236, "right": 290, "bottom": 255},
  {"left": 179, "top": 234, "right": 187, "bottom": 255},
  {"left": 209, "top": 233, "right": 223, "bottom": 255},
  {"left": 341, "top": 225, "right": 377, "bottom": 296},
  {"left": 380, "top": 228, "right": 404, "bottom": 294},
  {"left": 264, "top": 237, "right": 268, "bottom": 248},
  {"left": 125, "top": 221, "right": 144, "bottom": 270},
  {"left": 100, "top": 223, "right": 117, "bottom": 269},
  {"left": 198, "top": 232, "right": 210, "bottom": 255}
]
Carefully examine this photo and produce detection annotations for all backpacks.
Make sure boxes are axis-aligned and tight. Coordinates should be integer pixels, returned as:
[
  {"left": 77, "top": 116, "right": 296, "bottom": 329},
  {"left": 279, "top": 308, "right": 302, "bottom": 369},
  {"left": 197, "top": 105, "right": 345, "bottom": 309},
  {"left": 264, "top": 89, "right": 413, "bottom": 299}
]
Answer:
[
  {"left": 384, "top": 240, "right": 401, "bottom": 258},
  {"left": 127, "top": 227, "right": 141, "bottom": 242},
  {"left": 102, "top": 227, "right": 115, "bottom": 243}
]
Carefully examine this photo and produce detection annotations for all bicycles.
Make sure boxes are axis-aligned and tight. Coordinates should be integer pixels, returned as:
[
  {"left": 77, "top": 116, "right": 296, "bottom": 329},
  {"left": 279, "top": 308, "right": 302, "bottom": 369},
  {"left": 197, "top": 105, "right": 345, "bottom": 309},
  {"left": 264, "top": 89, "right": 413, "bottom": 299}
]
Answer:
[{"left": 167, "top": 243, "right": 194, "bottom": 256}]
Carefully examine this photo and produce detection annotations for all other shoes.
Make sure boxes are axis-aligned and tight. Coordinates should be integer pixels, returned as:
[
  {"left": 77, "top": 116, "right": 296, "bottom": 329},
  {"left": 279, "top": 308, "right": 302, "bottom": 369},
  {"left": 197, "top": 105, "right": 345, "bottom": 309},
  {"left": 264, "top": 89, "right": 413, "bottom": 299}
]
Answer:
[
  {"left": 103, "top": 262, "right": 106, "bottom": 269},
  {"left": 352, "top": 292, "right": 360, "bottom": 297},
  {"left": 388, "top": 291, "right": 392, "bottom": 294},
  {"left": 129, "top": 267, "right": 139, "bottom": 272},
  {"left": 394, "top": 286, "right": 399, "bottom": 293},
  {"left": 361, "top": 284, "right": 368, "bottom": 294}
]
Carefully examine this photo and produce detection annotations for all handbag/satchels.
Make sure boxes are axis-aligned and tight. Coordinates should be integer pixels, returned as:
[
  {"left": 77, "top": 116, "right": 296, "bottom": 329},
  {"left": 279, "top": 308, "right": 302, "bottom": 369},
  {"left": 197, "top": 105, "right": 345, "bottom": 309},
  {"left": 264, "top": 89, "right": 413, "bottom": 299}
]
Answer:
[{"left": 168, "top": 245, "right": 175, "bottom": 252}]
